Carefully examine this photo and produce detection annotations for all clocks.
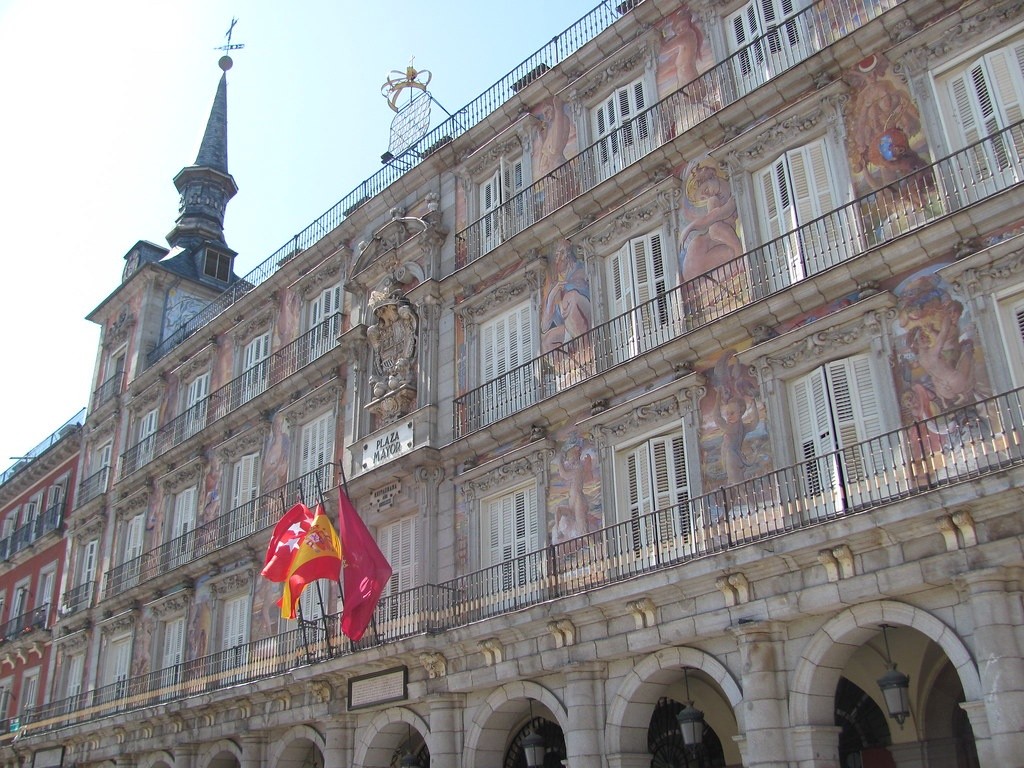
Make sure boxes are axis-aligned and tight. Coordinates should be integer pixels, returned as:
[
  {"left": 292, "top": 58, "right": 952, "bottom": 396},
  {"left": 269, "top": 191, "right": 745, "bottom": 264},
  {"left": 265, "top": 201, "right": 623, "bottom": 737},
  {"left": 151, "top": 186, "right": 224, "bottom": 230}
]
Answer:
[{"left": 121, "top": 247, "right": 139, "bottom": 283}]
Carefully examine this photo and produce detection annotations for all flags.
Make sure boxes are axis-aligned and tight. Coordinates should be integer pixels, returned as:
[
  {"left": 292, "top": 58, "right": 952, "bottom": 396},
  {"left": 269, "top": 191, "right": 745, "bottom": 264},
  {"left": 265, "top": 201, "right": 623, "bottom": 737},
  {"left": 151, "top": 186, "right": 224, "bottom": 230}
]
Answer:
[
  {"left": 277, "top": 503, "right": 342, "bottom": 620},
  {"left": 260, "top": 502, "right": 314, "bottom": 583},
  {"left": 337, "top": 486, "right": 392, "bottom": 642}
]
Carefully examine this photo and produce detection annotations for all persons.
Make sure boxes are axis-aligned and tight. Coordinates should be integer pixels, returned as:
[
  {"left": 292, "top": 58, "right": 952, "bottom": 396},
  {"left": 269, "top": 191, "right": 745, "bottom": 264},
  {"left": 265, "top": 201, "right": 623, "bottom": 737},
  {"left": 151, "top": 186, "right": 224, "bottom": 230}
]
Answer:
[
  {"left": 656, "top": 9, "right": 707, "bottom": 130},
  {"left": 260, "top": 415, "right": 290, "bottom": 510},
  {"left": 901, "top": 300, "right": 974, "bottom": 446},
  {"left": 678, "top": 164, "right": 743, "bottom": 289},
  {"left": 275, "top": 293, "right": 292, "bottom": 371},
  {"left": 712, "top": 385, "right": 760, "bottom": 521},
  {"left": 540, "top": 241, "right": 591, "bottom": 368},
  {"left": 548, "top": 455, "right": 591, "bottom": 550},
  {"left": 538, "top": 96, "right": 570, "bottom": 206},
  {"left": 131, "top": 336, "right": 235, "bottom": 699},
  {"left": 853, "top": 70, "right": 935, "bottom": 211}
]
